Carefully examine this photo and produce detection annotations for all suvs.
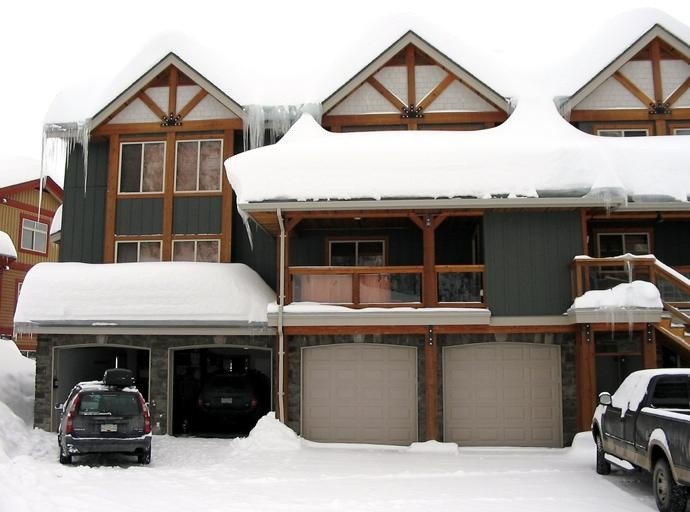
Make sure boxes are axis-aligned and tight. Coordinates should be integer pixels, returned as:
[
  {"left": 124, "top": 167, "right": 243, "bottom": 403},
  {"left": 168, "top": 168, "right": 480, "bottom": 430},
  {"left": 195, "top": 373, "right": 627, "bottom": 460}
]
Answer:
[
  {"left": 53, "top": 368, "right": 152, "bottom": 468},
  {"left": 198, "top": 369, "right": 258, "bottom": 415}
]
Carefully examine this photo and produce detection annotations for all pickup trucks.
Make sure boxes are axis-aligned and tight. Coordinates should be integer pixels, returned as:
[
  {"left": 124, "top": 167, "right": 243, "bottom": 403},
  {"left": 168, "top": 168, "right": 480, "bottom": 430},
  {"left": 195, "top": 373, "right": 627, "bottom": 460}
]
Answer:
[{"left": 590, "top": 367, "right": 690, "bottom": 512}]
